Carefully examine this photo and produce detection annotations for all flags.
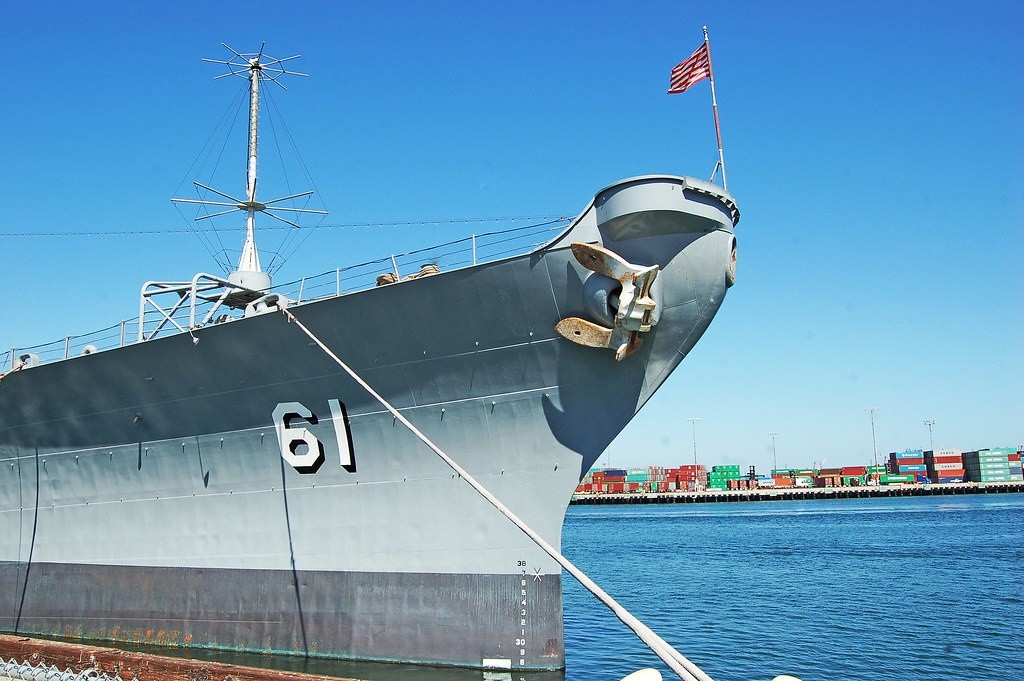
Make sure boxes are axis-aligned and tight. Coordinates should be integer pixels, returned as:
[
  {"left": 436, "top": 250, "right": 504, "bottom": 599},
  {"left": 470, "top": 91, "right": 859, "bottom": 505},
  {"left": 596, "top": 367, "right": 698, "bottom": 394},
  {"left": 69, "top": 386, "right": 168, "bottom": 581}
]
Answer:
[{"left": 667, "top": 41, "right": 712, "bottom": 93}]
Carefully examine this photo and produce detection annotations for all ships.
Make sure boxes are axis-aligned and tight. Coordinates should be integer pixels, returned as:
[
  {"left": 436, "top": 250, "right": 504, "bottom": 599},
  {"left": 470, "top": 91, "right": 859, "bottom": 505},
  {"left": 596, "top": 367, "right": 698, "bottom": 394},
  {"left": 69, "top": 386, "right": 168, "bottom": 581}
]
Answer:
[{"left": 0, "top": 24, "right": 745, "bottom": 680}]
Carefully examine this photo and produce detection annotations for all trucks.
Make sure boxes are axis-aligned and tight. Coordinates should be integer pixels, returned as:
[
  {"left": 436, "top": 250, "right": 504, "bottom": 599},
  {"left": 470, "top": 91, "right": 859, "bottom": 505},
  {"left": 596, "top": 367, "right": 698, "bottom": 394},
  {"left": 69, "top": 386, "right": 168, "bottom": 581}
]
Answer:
[{"left": 878, "top": 472, "right": 929, "bottom": 484}]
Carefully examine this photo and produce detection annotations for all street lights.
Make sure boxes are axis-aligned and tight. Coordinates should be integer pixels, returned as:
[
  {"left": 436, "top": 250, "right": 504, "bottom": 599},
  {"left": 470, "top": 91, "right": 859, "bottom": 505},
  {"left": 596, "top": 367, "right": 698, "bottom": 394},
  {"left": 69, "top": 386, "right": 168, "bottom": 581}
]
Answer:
[
  {"left": 768, "top": 432, "right": 782, "bottom": 488},
  {"left": 688, "top": 417, "right": 701, "bottom": 492},
  {"left": 923, "top": 420, "right": 935, "bottom": 451},
  {"left": 864, "top": 406, "right": 879, "bottom": 486}
]
